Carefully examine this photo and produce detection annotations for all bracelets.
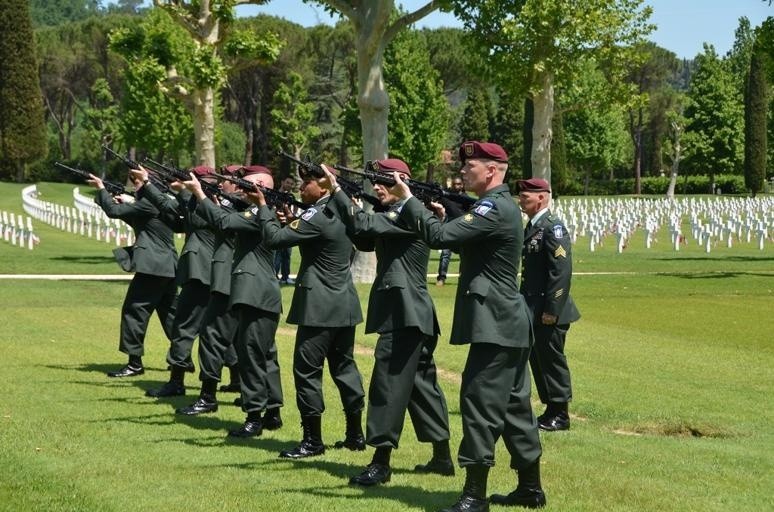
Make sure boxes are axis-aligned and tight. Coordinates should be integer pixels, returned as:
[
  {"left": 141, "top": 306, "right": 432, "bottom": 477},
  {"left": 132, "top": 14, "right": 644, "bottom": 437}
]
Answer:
[
  {"left": 143, "top": 180, "right": 149, "bottom": 184},
  {"left": 329, "top": 182, "right": 340, "bottom": 193}
]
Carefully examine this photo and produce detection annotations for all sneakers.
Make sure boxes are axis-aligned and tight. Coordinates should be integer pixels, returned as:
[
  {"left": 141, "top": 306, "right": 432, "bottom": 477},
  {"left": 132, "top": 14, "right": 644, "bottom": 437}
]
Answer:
[
  {"left": 436, "top": 279, "right": 444, "bottom": 288},
  {"left": 276, "top": 276, "right": 296, "bottom": 285}
]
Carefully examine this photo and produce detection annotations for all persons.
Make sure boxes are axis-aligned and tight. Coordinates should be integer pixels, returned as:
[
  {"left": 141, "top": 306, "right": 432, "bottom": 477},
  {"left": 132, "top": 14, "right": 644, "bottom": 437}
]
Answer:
[
  {"left": 515, "top": 179, "right": 581, "bottom": 431},
  {"left": 319, "top": 158, "right": 455, "bottom": 485},
  {"left": 248, "top": 165, "right": 365, "bottom": 459},
  {"left": 130, "top": 167, "right": 240, "bottom": 397},
  {"left": 183, "top": 165, "right": 283, "bottom": 438},
  {"left": 436, "top": 178, "right": 474, "bottom": 287},
  {"left": 390, "top": 141, "right": 545, "bottom": 512},
  {"left": 86, "top": 169, "right": 195, "bottom": 378},
  {"left": 171, "top": 164, "right": 246, "bottom": 416},
  {"left": 271, "top": 176, "right": 298, "bottom": 285}
]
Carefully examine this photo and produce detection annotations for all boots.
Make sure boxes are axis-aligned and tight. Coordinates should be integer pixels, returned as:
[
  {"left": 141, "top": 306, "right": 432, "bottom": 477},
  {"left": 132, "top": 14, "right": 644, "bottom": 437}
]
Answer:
[
  {"left": 168, "top": 357, "right": 195, "bottom": 372},
  {"left": 234, "top": 398, "right": 242, "bottom": 406},
  {"left": 220, "top": 367, "right": 240, "bottom": 392},
  {"left": 148, "top": 365, "right": 185, "bottom": 396},
  {"left": 415, "top": 440, "right": 454, "bottom": 475},
  {"left": 176, "top": 379, "right": 218, "bottom": 415},
  {"left": 231, "top": 412, "right": 262, "bottom": 436},
  {"left": 281, "top": 416, "right": 324, "bottom": 457},
  {"left": 438, "top": 465, "right": 489, "bottom": 512},
  {"left": 108, "top": 355, "right": 144, "bottom": 377},
  {"left": 490, "top": 457, "right": 545, "bottom": 508},
  {"left": 538, "top": 403, "right": 570, "bottom": 430},
  {"left": 335, "top": 410, "right": 365, "bottom": 450},
  {"left": 261, "top": 407, "right": 282, "bottom": 430},
  {"left": 350, "top": 446, "right": 392, "bottom": 486}
]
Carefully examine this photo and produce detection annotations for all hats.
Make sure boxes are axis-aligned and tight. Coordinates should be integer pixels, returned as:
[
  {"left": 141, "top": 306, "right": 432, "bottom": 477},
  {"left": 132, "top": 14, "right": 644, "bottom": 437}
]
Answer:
[
  {"left": 373, "top": 159, "right": 411, "bottom": 178},
  {"left": 516, "top": 178, "right": 550, "bottom": 193},
  {"left": 129, "top": 168, "right": 158, "bottom": 184},
  {"left": 242, "top": 165, "right": 272, "bottom": 178},
  {"left": 299, "top": 162, "right": 337, "bottom": 179},
  {"left": 224, "top": 165, "right": 243, "bottom": 175},
  {"left": 460, "top": 141, "right": 508, "bottom": 162},
  {"left": 191, "top": 166, "right": 215, "bottom": 175}
]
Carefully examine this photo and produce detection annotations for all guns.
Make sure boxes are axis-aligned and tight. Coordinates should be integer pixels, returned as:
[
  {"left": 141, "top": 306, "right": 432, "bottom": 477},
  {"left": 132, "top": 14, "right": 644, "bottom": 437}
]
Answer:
[
  {"left": 276, "top": 146, "right": 389, "bottom": 211},
  {"left": 207, "top": 169, "right": 311, "bottom": 211},
  {"left": 332, "top": 164, "right": 480, "bottom": 204},
  {"left": 101, "top": 144, "right": 176, "bottom": 195},
  {"left": 142, "top": 156, "right": 250, "bottom": 211},
  {"left": 54, "top": 160, "right": 135, "bottom": 198}
]
